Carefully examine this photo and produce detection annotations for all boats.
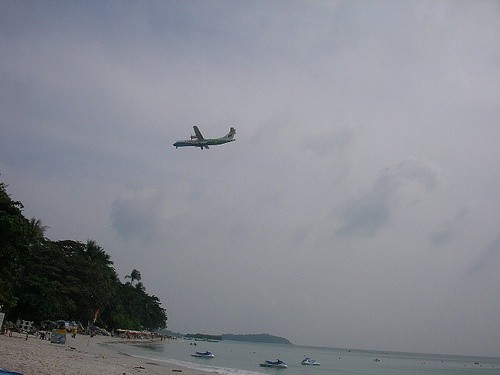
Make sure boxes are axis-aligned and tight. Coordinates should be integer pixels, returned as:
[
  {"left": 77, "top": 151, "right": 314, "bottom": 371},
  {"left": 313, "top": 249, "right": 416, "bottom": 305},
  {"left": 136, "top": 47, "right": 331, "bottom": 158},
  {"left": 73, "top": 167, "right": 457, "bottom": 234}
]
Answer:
[
  {"left": 301, "top": 361, "right": 321, "bottom": 366},
  {"left": 192, "top": 354, "right": 214, "bottom": 358},
  {"left": 260, "top": 361, "right": 288, "bottom": 368}
]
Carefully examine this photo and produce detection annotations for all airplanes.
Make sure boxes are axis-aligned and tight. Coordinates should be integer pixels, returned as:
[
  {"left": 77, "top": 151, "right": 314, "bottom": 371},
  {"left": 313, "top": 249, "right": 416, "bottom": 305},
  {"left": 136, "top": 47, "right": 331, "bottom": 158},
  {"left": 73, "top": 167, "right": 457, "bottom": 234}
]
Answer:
[{"left": 172, "top": 125, "right": 237, "bottom": 150}]
